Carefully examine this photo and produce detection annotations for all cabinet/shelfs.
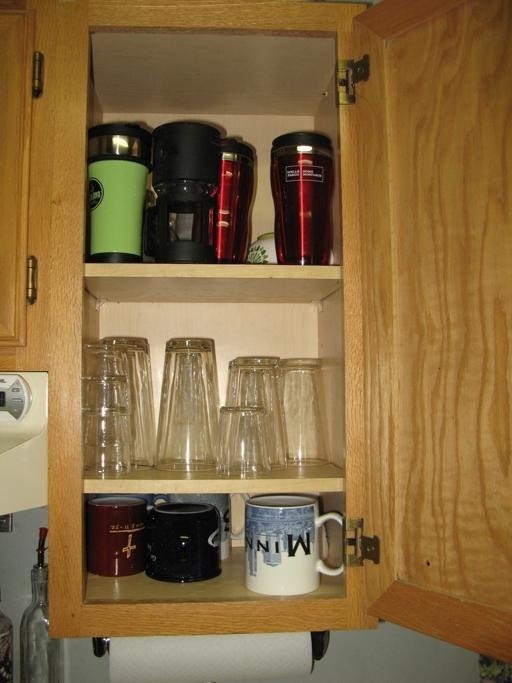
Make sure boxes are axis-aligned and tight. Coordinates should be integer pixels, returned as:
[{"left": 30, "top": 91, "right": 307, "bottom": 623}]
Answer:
[
  {"left": 47, "top": 0, "right": 512, "bottom": 666},
  {"left": 2, "top": 10, "right": 47, "bottom": 370}
]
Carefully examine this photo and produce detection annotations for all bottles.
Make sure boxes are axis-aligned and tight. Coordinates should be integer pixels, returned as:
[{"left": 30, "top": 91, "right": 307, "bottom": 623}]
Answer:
[
  {"left": 146, "top": 119, "right": 225, "bottom": 265},
  {"left": 85, "top": 121, "right": 153, "bottom": 266},
  {"left": 270, "top": 130, "right": 336, "bottom": 265},
  {"left": 19, "top": 527, "right": 61, "bottom": 683},
  {"left": 213, "top": 137, "right": 255, "bottom": 264}
]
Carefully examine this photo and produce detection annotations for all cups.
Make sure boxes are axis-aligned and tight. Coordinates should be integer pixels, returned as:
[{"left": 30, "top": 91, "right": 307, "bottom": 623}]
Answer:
[
  {"left": 84, "top": 492, "right": 250, "bottom": 584},
  {"left": 82, "top": 336, "right": 158, "bottom": 479},
  {"left": 218, "top": 355, "right": 332, "bottom": 479},
  {"left": 153, "top": 337, "right": 220, "bottom": 473},
  {"left": 243, "top": 493, "right": 344, "bottom": 597}
]
